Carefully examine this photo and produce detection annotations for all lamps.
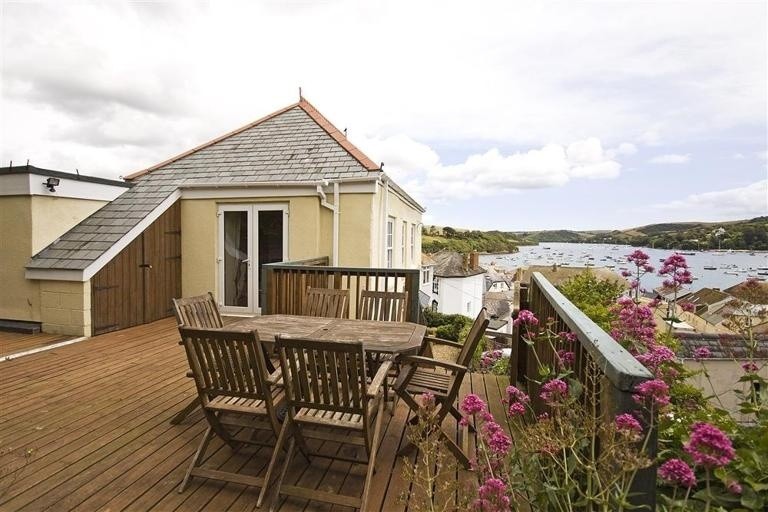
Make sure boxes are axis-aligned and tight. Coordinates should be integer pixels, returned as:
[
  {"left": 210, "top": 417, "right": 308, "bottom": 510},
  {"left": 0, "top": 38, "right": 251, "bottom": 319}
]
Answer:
[{"left": 42, "top": 176, "right": 59, "bottom": 193}]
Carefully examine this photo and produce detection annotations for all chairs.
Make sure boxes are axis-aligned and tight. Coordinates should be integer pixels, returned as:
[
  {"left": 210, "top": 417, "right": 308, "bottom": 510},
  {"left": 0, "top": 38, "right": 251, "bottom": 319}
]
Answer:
[
  {"left": 178, "top": 323, "right": 290, "bottom": 507},
  {"left": 270, "top": 333, "right": 393, "bottom": 510},
  {"left": 357, "top": 290, "right": 408, "bottom": 321},
  {"left": 170, "top": 291, "right": 225, "bottom": 426},
  {"left": 301, "top": 286, "right": 348, "bottom": 318},
  {"left": 395, "top": 307, "right": 489, "bottom": 469}
]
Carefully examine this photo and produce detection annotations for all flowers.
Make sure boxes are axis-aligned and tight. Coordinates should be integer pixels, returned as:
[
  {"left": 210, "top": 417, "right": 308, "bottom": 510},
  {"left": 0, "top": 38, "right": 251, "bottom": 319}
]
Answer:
[{"left": 391, "top": 248, "right": 768, "bottom": 511}]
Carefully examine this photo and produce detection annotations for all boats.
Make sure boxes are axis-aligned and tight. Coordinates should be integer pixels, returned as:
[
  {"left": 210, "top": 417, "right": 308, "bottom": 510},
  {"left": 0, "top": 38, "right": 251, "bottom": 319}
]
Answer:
[
  {"left": 676, "top": 252, "right": 696, "bottom": 255},
  {"left": 491, "top": 247, "right": 646, "bottom": 275},
  {"left": 704, "top": 263, "right": 768, "bottom": 281}
]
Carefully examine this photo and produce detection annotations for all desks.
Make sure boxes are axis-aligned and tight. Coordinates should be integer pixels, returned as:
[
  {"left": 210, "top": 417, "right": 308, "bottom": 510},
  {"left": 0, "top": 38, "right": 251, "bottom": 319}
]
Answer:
[{"left": 221, "top": 314, "right": 427, "bottom": 416}]
